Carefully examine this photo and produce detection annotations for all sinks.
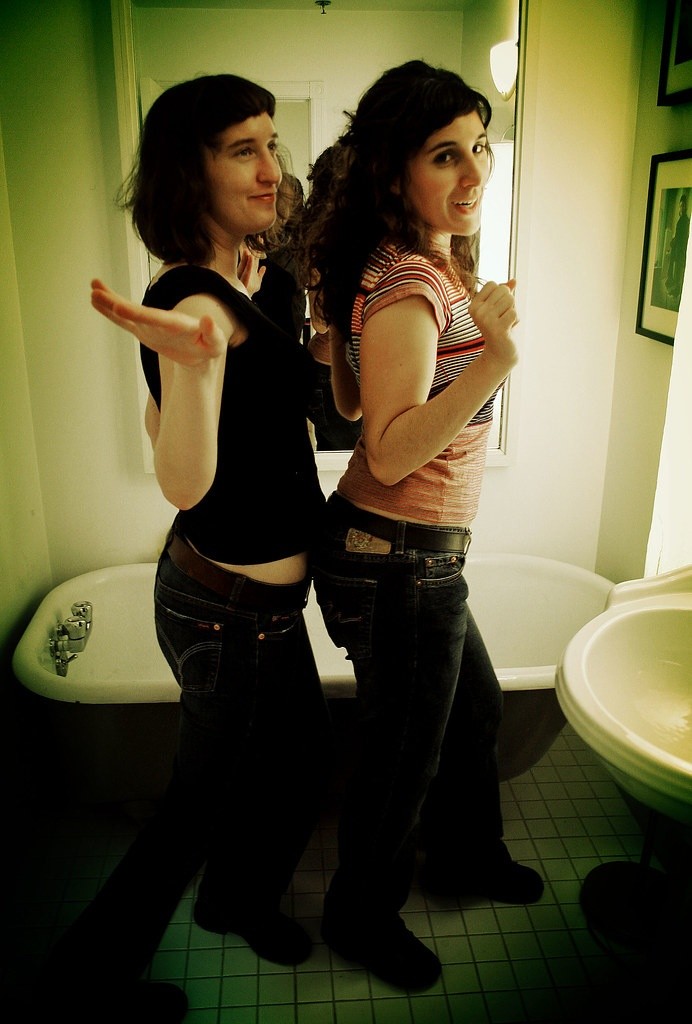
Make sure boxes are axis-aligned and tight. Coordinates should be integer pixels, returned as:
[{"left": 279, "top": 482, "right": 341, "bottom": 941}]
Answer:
[{"left": 555, "top": 566, "right": 692, "bottom": 826}]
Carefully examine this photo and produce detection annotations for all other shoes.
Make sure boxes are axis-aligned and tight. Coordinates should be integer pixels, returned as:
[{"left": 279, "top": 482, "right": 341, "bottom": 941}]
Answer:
[
  {"left": 319, "top": 892, "right": 442, "bottom": 993},
  {"left": 31, "top": 975, "right": 188, "bottom": 1024},
  {"left": 193, "top": 895, "right": 313, "bottom": 966},
  {"left": 416, "top": 840, "right": 544, "bottom": 904}
]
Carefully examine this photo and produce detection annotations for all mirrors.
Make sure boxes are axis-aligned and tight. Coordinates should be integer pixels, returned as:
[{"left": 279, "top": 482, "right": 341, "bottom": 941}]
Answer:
[{"left": 109, "top": 0, "right": 523, "bottom": 475}]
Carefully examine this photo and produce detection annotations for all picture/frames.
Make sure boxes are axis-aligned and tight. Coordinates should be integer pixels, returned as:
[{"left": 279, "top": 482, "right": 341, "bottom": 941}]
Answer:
[
  {"left": 636, "top": 149, "right": 692, "bottom": 348},
  {"left": 656, "top": 0, "right": 692, "bottom": 107}
]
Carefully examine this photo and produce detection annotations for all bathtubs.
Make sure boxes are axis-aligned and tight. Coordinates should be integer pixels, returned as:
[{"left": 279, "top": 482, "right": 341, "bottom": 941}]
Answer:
[{"left": 14, "top": 554, "right": 616, "bottom": 704}]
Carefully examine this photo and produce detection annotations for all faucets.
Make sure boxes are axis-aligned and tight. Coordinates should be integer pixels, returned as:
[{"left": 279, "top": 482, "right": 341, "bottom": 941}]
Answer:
[{"left": 57, "top": 616, "right": 91, "bottom": 636}]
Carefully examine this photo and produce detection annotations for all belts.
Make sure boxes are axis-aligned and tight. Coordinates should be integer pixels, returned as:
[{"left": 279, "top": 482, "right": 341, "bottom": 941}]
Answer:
[
  {"left": 325, "top": 490, "right": 469, "bottom": 553},
  {"left": 166, "top": 531, "right": 312, "bottom": 610}
]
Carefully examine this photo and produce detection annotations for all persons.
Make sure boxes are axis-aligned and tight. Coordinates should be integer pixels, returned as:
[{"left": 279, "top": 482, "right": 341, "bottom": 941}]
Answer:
[
  {"left": 285, "top": 59, "right": 545, "bottom": 993},
  {"left": 255, "top": 146, "right": 366, "bottom": 451},
  {"left": 51, "top": 72, "right": 328, "bottom": 1024}
]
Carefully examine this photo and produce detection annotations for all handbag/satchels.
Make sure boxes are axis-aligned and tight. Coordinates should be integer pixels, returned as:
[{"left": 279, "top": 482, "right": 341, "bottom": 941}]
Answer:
[{"left": 302, "top": 361, "right": 362, "bottom": 451}]
